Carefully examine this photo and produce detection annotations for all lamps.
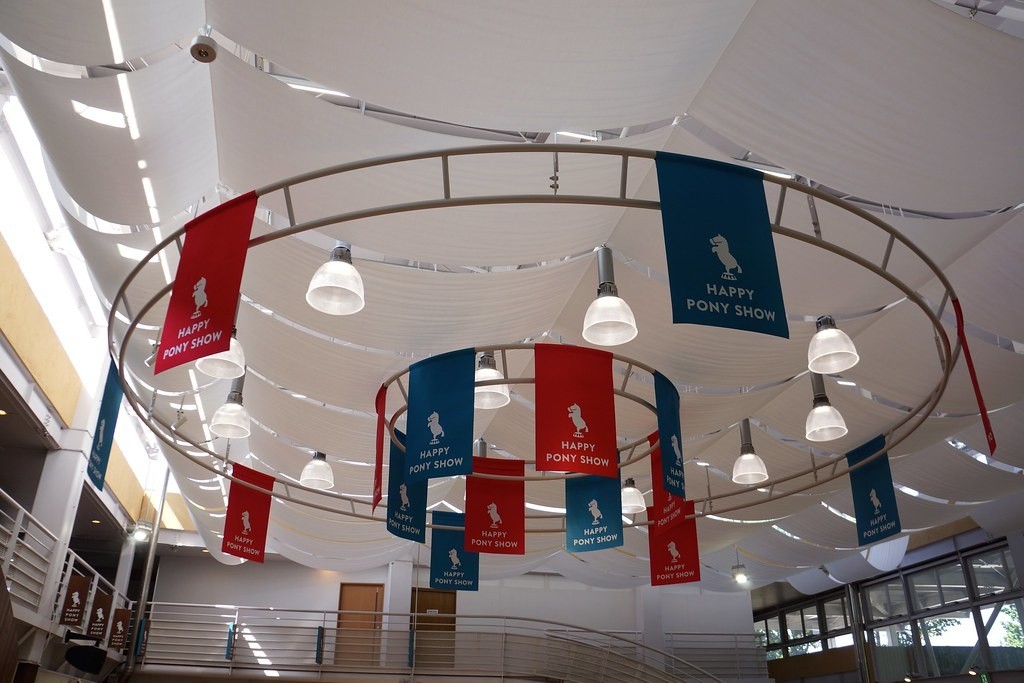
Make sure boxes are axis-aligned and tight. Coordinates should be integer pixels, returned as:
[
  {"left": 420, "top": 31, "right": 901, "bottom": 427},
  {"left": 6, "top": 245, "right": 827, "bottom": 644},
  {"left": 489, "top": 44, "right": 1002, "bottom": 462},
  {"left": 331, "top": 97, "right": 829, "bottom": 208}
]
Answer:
[
  {"left": 621, "top": 478, "right": 646, "bottom": 513},
  {"left": 806, "top": 371, "right": 848, "bottom": 442},
  {"left": 968, "top": 663, "right": 982, "bottom": 677},
  {"left": 731, "top": 419, "right": 768, "bottom": 484},
  {"left": 305, "top": 241, "right": 365, "bottom": 316},
  {"left": 208, "top": 364, "right": 251, "bottom": 438},
  {"left": 904, "top": 671, "right": 920, "bottom": 682},
  {"left": 808, "top": 315, "right": 859, "bottom": 374},
  {"left": 299, "top": 452, "right": 334, "bottom": 489},
  {"left": 190, "top": 36, "right": 218, "bottom": 63},
  {"left": 474, "top": 351, "right": 511, "bottom": 409},
  {"left": 582, "top": 244, "right": 638, "bottom": 347},
  {"left": 195, "top": 292, "right": 246, "bottom": 380}
]
